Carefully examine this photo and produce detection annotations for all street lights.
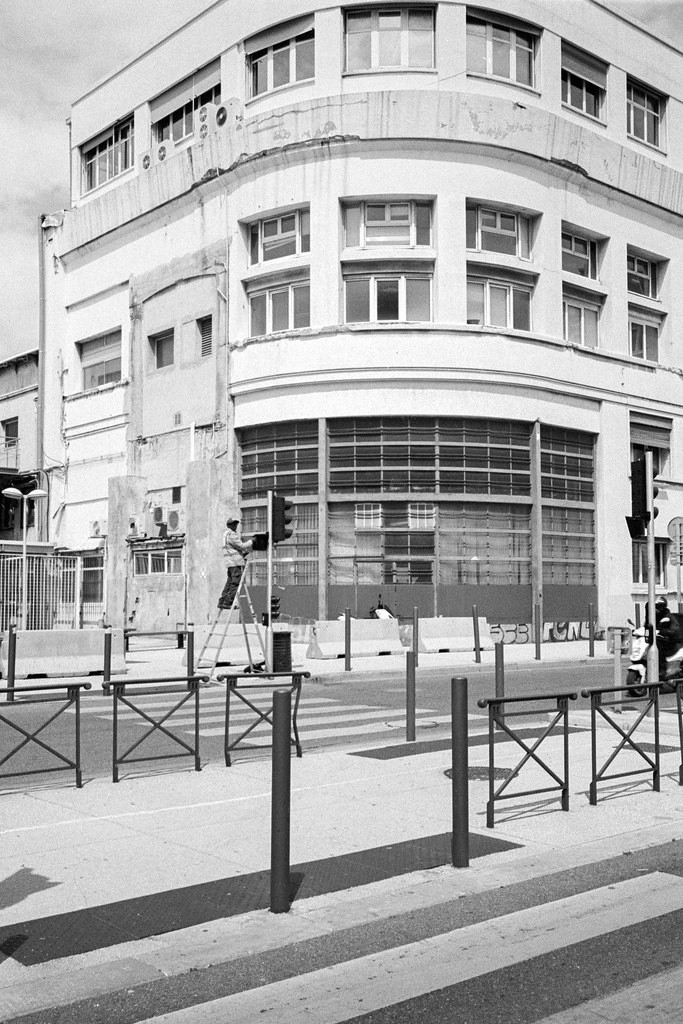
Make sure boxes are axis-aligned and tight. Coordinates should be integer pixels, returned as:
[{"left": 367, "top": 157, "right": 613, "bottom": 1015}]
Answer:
[
  {"left": 1, "top": 487, "right": 49, "bottom": 631},
  {"left": 36, "top": 210, "right": 68, "bottom": 544}
]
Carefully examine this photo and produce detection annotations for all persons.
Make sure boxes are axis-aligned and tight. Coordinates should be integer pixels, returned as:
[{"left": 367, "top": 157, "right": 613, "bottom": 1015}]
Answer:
[
  {"left": 655, "top": 596, "right": 680, "bottom": 678},
  {"left": 217, "top": 518, "right": 256, "bottom": 609}
]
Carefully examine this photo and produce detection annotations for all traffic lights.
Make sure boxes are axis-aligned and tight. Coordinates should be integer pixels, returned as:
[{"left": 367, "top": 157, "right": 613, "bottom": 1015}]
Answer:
[
  {"left": 631, "top": 460, "right": 658, "bottom": 524},
  {"left": 253, "top": 532, "right": 268, "bottom": 551},
  {"left": 270, "top": 595, "right": 280, "bottom": 619},
  {"left": 271, "top": 494, "right": 293, "bottom": 544}
]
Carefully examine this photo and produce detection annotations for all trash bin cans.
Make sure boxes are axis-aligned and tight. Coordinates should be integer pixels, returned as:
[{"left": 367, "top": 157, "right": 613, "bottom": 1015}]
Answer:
[{"left": 273, "top": 631, "right": 293, "bottom": 672}]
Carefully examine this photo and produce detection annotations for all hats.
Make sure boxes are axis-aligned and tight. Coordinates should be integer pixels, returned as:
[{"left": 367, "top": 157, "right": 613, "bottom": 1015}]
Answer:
[
  {"left": 227, "top": 518, "right": 239, "bottom": 525},
  {"left": 654, "top": 596, "right": 667, "bottom": 605}
]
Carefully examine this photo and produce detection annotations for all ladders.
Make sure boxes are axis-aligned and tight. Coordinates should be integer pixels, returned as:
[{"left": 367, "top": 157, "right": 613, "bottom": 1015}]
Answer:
[{"left": 192, "top": 558, "right": 274, "bottom": 687}]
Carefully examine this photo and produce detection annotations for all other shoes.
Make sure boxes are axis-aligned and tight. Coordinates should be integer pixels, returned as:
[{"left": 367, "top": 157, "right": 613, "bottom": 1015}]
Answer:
[
  {"left": 217, "top": 604, "right": 223, "bottom": 608},
  {"left": 222, "top": 604, "right": 237, "bottom": 609},
  {"left": 659, "top": 670, "right": 666, "bottom": 676}
]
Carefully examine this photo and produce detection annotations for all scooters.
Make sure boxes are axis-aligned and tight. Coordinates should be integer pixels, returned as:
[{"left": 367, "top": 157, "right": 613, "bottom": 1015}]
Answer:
[
  {"left": 336, "top": 594, "right": 395, "bottom": 621},
  {"left": 626, "top": 616, "right": 683, "bottom": 697}
]
